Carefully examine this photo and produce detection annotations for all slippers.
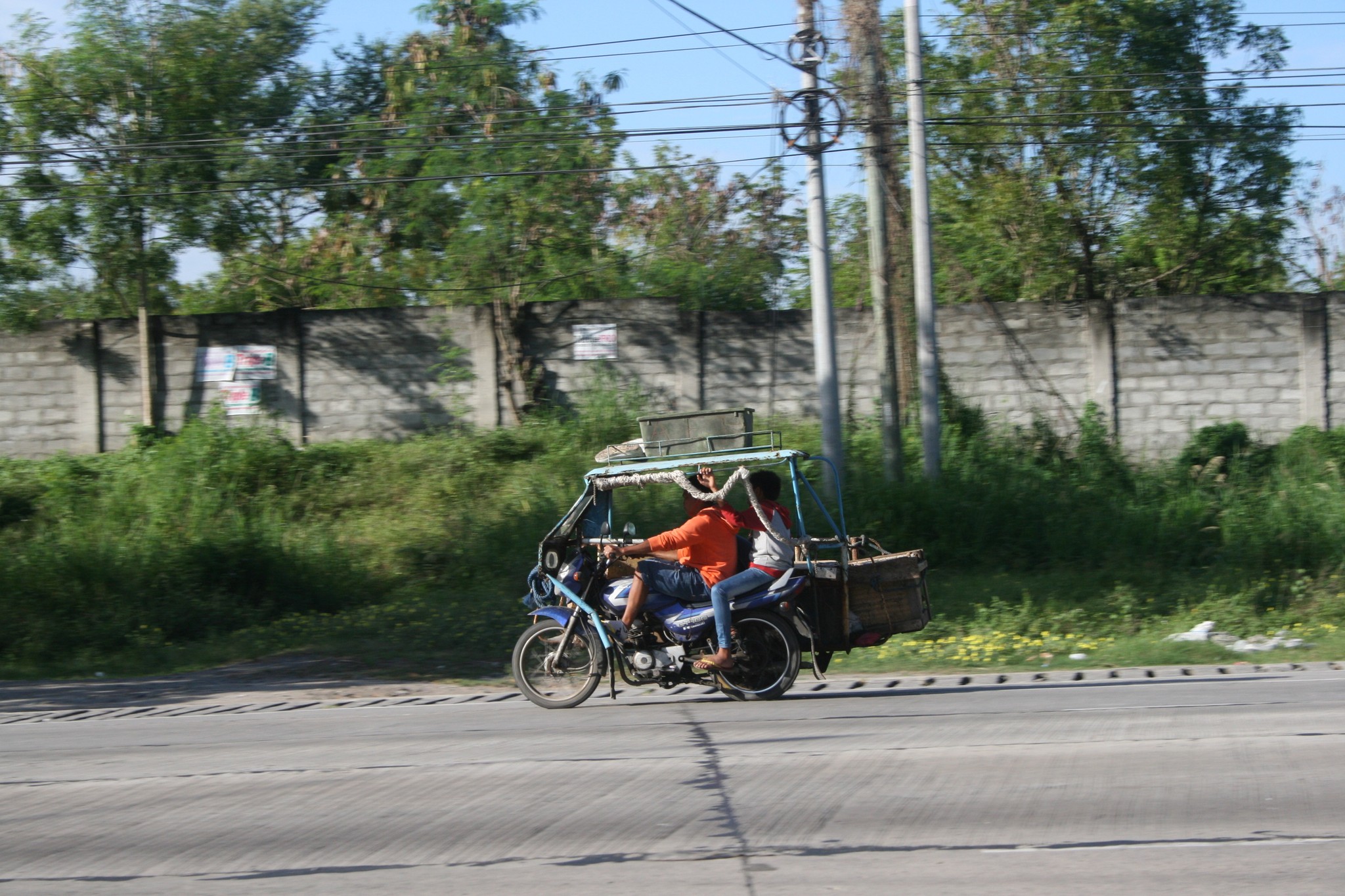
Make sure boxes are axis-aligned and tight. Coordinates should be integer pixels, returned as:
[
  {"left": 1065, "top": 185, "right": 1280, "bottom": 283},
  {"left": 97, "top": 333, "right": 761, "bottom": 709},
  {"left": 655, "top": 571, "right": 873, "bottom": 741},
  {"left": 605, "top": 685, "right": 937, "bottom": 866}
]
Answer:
[{"left": 693, "top": 657, "right": 739, "bottom": 674}]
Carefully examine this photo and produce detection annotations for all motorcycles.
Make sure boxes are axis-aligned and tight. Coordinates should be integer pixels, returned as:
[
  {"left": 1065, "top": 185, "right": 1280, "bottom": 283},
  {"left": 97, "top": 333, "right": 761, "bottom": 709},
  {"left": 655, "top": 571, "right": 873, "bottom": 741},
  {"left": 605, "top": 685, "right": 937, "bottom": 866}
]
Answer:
[{"left": 510, "top": 433, "right": 892, "bottom": 709}]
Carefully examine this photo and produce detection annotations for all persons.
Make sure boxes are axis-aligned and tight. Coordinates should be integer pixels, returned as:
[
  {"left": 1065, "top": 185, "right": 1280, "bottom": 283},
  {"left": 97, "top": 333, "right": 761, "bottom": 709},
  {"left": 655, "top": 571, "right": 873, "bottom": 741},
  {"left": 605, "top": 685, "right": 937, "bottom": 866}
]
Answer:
[
  {"left": 599, "top": 475, "right": 742, "bottom": 645},
  {"left": 692, "top": 470, "right": 796, "bottom": 673}
]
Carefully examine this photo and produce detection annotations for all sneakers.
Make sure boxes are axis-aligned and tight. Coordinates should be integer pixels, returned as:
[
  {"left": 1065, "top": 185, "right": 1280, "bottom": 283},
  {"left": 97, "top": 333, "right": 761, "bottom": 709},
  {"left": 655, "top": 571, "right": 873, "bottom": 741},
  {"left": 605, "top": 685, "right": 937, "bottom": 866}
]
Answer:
[
  {"left": 621, "top": 438, "right": 645, "bottom": 454},
  {"left": 587, "top": 619, "right": 629, "bottom": 643},
  {"left": 594, "top": 442, "right": 648, "bottom": 464}
]
